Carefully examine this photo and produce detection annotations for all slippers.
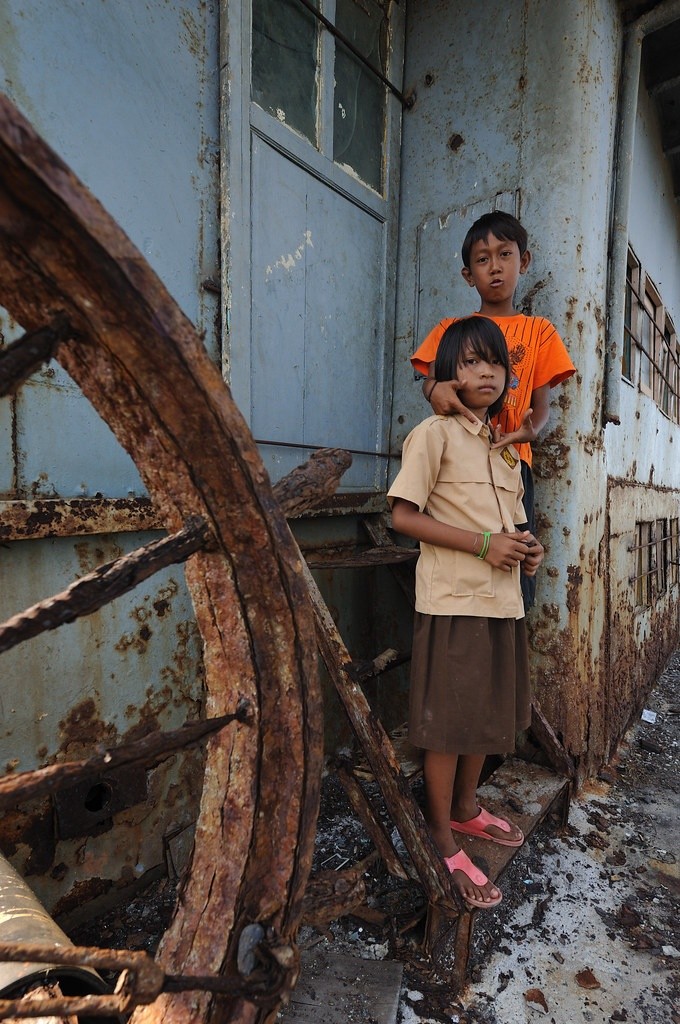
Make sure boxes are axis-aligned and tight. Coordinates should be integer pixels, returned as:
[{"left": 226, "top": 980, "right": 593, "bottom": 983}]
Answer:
[{"left": 435, "top": 804, "right": 524, "bottom": 908}]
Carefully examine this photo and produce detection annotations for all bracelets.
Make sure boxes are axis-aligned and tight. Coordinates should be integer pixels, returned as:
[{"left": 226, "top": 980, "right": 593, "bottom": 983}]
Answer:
[
  {"left": 471, "top": 533, "right": 481, "bottom": 557},
  {"left": 476, "top": 531, "right": 492, "bottom": 560},
  {"left": 427, "top": 380, "right": 438, "bottom": 403}
]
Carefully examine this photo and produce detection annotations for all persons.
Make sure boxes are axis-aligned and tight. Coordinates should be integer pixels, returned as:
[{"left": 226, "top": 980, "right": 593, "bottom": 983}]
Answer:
[
  {"left": 408, "top": 211, "right": 574, "bottom": 790},
  {"left": 385, "top": 315, "right": 545, "bottom": 908}
]
